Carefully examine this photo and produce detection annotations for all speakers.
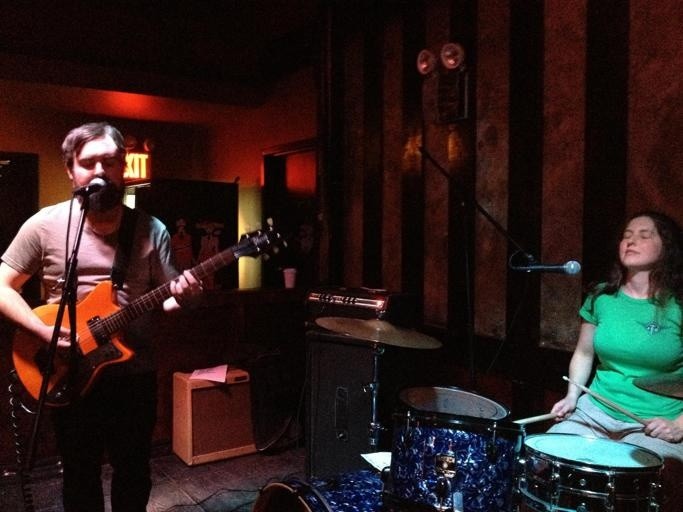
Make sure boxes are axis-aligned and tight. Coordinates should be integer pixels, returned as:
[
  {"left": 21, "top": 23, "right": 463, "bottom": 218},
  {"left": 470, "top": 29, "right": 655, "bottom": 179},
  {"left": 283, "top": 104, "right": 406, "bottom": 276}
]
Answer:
[{"left": 303, "top": 330, "right": 415, "bottom": 481}]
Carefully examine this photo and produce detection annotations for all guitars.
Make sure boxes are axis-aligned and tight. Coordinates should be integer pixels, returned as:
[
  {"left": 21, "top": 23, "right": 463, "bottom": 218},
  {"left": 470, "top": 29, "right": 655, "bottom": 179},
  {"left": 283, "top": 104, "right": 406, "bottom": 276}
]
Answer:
[{"left": 12, "top": 218, "right": 289, "bottom": 409}]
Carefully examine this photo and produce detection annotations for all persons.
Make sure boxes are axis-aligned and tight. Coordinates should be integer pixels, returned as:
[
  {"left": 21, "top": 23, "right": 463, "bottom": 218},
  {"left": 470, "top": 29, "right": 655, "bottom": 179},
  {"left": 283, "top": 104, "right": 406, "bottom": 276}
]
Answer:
[
  {"left": 0, "top": 123, "right": 204, "bottom": 510},
  {"left": 545, "top": 206, "right": 682, "bottom": 512}
]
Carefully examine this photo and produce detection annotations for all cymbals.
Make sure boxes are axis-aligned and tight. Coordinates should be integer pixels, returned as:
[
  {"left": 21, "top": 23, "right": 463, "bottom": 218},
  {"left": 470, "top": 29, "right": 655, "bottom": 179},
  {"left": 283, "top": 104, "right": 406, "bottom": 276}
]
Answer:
[
  {"left": 314, "top": 316, "right": 443, "bottom": 349},
  {"left": 633, "top": 369, "right": 683, "bottom": 401}
]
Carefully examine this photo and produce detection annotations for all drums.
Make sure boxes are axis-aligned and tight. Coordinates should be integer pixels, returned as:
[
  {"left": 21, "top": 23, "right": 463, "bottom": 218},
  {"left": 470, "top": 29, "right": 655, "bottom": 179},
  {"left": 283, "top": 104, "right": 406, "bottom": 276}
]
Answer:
[
  {"left": 514, "top": 432, "right": 668, "bottom": 511},
  {"left": 382, "top": 407, "right": 527, "bottom": 512},
  {"left": 253, "top": 463, "right": 386, "bottom": 512},
  {"left": 392, "top": 385, "right": 512, "bottom": 429}
]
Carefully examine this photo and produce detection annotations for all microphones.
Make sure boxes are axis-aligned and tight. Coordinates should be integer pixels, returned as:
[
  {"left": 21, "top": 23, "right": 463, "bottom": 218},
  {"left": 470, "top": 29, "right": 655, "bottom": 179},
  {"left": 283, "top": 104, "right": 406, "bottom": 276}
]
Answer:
[
  {"left": 517, "top": 260, "right": 582, "bottom": 276},
  {"left": 71, "top": 178, "right": 106, "bottom": 196}
]
